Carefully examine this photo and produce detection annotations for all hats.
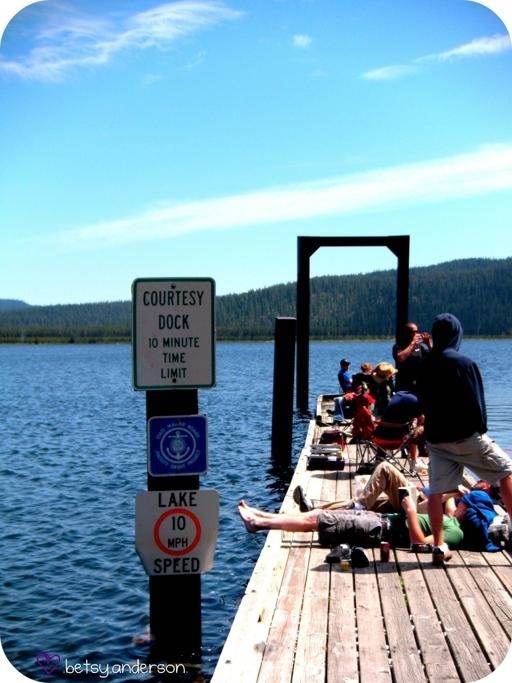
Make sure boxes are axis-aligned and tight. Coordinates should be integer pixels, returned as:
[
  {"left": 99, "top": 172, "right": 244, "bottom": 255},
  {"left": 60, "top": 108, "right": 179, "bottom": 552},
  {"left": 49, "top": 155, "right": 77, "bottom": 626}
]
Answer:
[
  {"left": 372, "top": 362, "right": 399, "bottom": 384},
  {"left": 340, "top": 358, "right": 351, "bottom": 366}
]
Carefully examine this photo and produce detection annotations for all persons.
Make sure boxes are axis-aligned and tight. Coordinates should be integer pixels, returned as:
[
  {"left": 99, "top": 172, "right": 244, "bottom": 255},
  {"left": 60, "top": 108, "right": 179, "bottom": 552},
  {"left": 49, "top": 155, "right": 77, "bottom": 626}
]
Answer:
[
  {"left": 337, "top": 321, "right": 434, "bottom": 477},
  {"left": 424, "top": 311, "right": 511, "bottom": 565},
  {"left": 235, "top": 489, "right": 497, "bottom": 547},
  {"left": 291, "top": 460, "right": 499, "bottom": 516}
]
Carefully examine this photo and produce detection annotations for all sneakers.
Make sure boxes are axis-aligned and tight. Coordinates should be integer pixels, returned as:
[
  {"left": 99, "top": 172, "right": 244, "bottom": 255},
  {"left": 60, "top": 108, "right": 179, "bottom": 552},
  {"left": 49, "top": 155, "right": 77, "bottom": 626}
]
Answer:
[
  {"left": 294, "top": 486, "right": 314, "bottom": 512},
  {"left": 433, "top": 543, "right": 452, "bottom": 567},
  {"left": 325, "top": 544, "right": 369, "bottom": 569}
]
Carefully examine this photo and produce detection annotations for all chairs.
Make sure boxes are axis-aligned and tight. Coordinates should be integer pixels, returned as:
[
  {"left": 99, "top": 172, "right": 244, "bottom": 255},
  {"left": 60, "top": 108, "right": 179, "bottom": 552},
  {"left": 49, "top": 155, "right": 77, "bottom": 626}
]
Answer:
[{"left": 334, "top": 396, "right": 418, "bottom": 477}]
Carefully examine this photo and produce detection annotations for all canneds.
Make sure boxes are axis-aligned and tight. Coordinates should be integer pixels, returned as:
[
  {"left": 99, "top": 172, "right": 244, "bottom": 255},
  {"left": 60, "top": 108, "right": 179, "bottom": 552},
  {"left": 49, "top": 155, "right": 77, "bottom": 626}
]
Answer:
[{"left": 380, "top": 542, "right": 390, "bottom": 563}]
